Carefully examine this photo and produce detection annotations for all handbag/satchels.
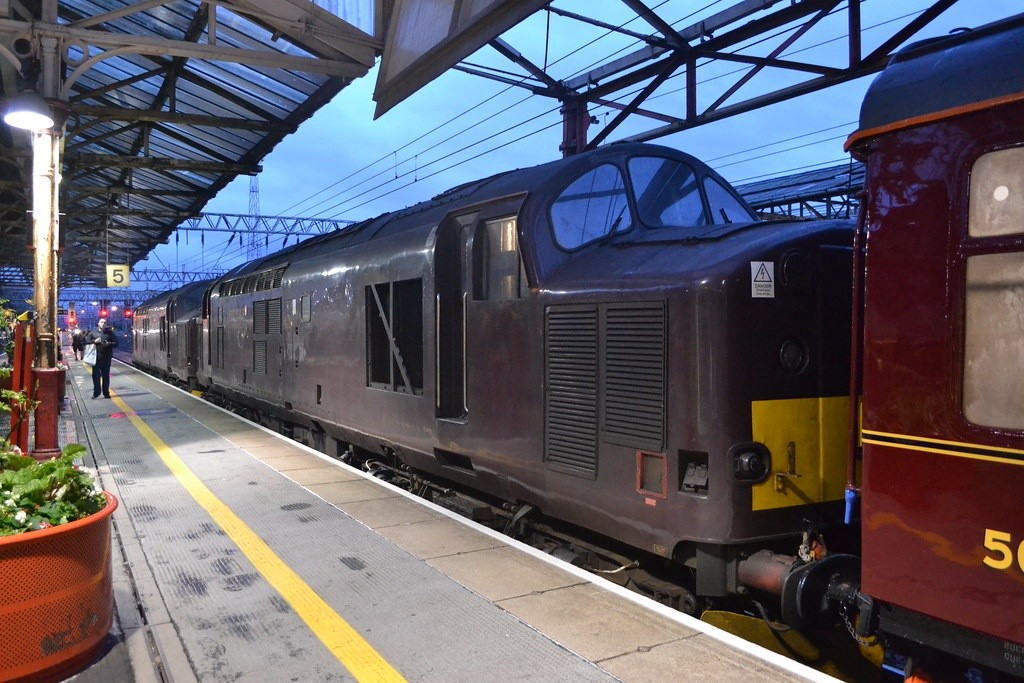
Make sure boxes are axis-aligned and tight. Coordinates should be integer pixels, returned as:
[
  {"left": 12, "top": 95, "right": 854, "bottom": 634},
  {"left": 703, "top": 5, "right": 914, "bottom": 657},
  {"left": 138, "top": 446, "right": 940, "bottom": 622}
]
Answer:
[{"left": 83, "top": 332, "right": 97, "bottom": 366}]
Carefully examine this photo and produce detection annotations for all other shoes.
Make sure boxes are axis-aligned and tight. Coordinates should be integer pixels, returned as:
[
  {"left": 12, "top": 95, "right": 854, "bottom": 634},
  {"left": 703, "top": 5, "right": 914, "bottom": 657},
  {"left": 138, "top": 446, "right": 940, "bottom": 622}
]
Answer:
[
  {"left": 104, "top": 394, "right": 111, "bottom": 398},
  {"left": 92, "top": 393, "right": 101, "bottom": 399}
]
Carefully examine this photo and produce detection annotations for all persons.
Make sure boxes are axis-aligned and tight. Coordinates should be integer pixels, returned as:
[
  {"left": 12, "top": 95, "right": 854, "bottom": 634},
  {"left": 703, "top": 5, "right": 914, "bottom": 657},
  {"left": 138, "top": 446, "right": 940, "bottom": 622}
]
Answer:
[
  {"left": 85, "top": 318, "right": 119, "bottom": 399},
  {"left": 71, "top": 326, "right": 91, "bottom": 361}
]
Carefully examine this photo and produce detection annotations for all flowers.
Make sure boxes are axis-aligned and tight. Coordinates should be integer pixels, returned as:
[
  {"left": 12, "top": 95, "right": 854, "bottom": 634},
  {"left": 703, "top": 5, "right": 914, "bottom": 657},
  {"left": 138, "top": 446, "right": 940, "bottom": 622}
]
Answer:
[{"left": 0, "top": 300, "right": 108, "bottom": 537}]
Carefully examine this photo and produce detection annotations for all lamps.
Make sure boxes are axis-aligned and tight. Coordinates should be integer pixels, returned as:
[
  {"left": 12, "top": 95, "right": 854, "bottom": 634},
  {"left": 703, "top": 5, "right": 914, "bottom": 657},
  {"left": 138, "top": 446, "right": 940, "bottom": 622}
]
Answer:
[{"left": 4, "top": 55, "right": 54, "bottom": 129}]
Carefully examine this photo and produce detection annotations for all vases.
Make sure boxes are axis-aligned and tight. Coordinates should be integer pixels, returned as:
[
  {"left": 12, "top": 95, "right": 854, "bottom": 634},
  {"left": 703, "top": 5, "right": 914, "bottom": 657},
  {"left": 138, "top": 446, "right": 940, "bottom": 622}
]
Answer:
[{"left": 0, "top": 490, "right": 118, "bottom": 683}]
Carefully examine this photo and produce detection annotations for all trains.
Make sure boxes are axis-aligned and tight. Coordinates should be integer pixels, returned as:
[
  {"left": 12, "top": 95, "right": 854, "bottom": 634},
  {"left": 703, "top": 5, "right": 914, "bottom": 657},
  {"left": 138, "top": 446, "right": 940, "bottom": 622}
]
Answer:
[{"left": 129, "top": 8, "right": 1023, "bottom": 683}]
